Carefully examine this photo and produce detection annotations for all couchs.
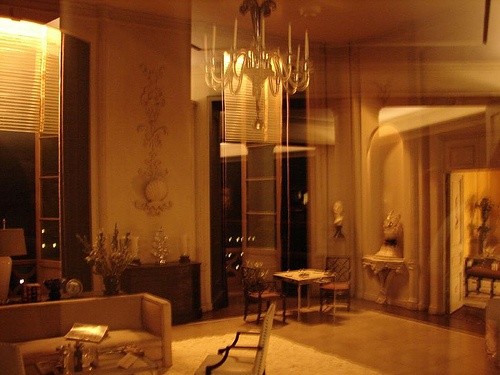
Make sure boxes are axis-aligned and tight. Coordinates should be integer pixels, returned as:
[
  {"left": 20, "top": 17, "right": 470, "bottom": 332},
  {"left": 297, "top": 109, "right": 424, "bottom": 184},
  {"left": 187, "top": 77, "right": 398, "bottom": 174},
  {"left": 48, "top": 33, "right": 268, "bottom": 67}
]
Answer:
[{"left": 0, "top": 293, "right": 172, "bottom": 375}]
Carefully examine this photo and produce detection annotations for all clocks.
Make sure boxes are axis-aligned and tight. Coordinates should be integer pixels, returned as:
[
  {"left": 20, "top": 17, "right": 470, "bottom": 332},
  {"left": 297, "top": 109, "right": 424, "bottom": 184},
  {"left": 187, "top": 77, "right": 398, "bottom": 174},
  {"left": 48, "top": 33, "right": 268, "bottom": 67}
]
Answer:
[{"left": 66, "top": 280, "right": 84, "bottom": 297}]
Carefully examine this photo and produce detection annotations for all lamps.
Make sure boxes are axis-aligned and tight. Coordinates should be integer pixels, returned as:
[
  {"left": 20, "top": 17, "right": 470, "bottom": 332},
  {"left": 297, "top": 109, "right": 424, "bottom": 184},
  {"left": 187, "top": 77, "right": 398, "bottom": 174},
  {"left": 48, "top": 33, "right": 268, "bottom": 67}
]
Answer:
[
  {"left": 202, "top": 0, "right": 310, "bottom": 130},
  {"left": 0, "top": 218, "right": 27, "bottom": 305}
]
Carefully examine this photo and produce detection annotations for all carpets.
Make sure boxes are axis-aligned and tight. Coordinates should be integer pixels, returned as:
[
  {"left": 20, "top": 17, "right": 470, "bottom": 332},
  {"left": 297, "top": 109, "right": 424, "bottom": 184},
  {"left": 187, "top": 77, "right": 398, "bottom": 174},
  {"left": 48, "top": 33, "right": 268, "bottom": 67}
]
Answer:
[{"left": 134, "top": 329, "right": 376, "bottom": 375}]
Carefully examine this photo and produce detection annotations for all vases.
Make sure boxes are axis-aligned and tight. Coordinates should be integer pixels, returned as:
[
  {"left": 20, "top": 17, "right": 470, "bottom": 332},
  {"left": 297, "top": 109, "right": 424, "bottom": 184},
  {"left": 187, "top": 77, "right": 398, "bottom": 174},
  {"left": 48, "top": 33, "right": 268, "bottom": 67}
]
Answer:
[{"left": 104, "top": 277, "right": 118, "bottom": 297}]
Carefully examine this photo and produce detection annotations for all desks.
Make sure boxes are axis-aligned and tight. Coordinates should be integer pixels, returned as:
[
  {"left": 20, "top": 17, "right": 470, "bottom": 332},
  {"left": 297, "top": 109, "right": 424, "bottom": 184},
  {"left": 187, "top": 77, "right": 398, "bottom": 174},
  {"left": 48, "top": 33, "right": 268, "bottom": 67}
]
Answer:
[
  {"left": 273, "top": 269, "right": 337, "bottom": 322},
  {"left": 122, "top": 261, "right": 201, "bottom": 326}
]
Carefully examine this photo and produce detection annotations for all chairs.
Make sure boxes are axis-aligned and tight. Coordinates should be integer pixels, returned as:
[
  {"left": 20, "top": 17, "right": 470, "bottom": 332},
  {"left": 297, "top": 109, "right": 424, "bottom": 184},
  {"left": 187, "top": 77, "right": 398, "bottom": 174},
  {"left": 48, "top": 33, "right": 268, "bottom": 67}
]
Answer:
[
  {"left": 241, "top": 266, "right": 287, "bottom": 326},
  {"left": 319, "top": 256, "right": 350, "bottom": 314},
  {"left": 191, "top": 304, "right": 275, "bottom": 375}
]
